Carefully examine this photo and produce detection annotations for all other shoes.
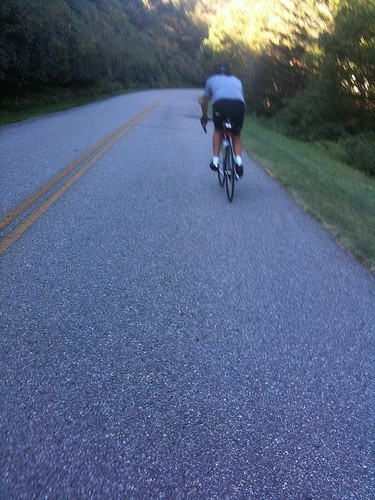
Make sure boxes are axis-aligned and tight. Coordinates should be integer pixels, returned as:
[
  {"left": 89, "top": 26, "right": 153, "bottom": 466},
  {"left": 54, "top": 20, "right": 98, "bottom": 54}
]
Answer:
[
  {"left": 235, "top": 163, "right": 244, "bottom": 175},
  {"left": 209, "top": 160, "right": 219, "bottom": 170}
]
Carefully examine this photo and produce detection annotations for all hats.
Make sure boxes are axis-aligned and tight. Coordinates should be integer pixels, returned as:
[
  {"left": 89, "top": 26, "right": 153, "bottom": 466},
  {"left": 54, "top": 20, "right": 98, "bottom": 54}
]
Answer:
[{"left": 213, "top": 63, "right": 232, "bottom": 75}]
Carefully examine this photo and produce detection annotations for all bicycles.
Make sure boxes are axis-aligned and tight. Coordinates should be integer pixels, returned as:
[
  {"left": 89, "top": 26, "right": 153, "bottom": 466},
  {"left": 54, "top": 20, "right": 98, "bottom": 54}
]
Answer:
[{"left": 201, "top": 116, "right": 241, "bottom": 204}]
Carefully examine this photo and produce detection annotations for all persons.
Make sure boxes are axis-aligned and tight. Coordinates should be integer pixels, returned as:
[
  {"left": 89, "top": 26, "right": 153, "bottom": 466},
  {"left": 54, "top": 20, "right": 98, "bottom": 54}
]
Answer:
[{"left": 200, "top": 65, "right": 246, "bottom": 178}]
